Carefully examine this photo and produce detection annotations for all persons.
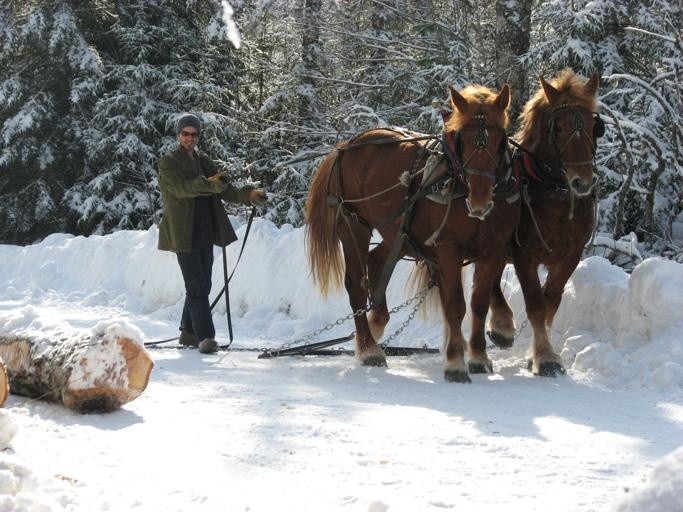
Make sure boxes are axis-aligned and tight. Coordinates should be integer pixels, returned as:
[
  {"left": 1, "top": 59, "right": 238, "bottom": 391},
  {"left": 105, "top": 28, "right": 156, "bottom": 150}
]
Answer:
[{"left": 154, "top": 113, "right": 269, "bottom": 354}]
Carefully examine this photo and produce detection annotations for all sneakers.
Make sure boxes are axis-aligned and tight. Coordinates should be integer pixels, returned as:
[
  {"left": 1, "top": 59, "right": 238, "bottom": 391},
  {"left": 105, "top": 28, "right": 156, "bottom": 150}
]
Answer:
[
  {"left": 198, "top": 338, "right": 218, "bottom": 354},
  {"left": 179, "top": 330, "right": 200, "bottom": 347}
]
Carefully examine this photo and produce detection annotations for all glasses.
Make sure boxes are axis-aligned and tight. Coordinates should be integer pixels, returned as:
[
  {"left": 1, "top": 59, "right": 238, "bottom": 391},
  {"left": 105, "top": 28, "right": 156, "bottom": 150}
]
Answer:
[{"left": 179, "top": 130, "right": 199, "bottom": 137}]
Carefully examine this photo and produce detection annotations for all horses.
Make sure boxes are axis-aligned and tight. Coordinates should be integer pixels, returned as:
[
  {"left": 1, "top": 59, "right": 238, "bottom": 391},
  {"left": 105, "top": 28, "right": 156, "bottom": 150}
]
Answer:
[
  {"left": 302, "top": 83, "right": 522, "bottom": 384},
  {"left": 404, "top": 67, "right": 599, "bottom": 377}
]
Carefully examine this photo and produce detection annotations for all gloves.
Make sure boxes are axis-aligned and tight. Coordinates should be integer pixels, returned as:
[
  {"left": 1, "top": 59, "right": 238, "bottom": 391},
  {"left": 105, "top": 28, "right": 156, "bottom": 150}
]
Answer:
[
  {"left": 208, "top": 171, "right": 231, "bottom": 195},
  {"left": 249, "top": 189, "right": 269, "bottom": 209}
]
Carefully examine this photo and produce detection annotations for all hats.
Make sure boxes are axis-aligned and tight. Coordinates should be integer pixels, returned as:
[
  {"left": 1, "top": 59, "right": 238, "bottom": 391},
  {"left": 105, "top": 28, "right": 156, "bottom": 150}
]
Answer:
[{"left": 175, "top": 114, "right": 201, "bottom": 137}]
